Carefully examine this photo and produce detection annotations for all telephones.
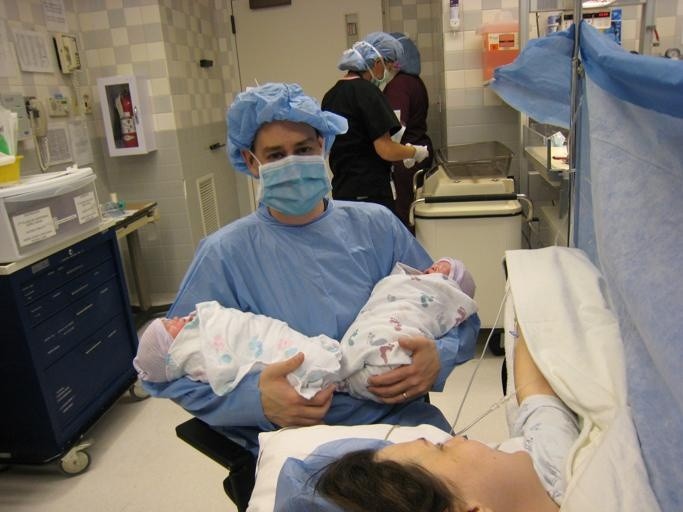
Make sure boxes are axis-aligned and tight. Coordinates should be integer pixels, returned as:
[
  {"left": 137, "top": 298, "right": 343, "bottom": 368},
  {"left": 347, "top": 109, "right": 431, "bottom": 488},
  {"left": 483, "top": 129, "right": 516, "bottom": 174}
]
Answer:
[{"left": 25, "top": 99, "right": 47, "bottom": 138}]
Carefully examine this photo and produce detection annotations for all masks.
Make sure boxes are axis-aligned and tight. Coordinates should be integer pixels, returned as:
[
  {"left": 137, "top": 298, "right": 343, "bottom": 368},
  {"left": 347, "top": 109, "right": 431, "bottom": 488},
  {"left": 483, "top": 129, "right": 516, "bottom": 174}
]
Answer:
[
  {"left": 257, "top": 155, "right": 335, "bottom": 215},
  {"left": 370, "top": 68, "right": 394, "bottom": 86}
]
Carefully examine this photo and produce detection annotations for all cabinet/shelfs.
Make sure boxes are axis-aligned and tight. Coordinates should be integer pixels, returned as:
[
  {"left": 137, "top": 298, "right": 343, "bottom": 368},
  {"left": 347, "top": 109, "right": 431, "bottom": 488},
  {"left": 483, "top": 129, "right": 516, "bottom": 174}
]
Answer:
[{"left": 0, "top": 218, "right": 143, "bottom": 467}]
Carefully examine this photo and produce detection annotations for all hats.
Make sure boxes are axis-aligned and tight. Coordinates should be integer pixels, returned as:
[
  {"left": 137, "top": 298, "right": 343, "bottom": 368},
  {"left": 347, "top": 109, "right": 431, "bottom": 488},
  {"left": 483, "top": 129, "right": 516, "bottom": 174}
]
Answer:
[
  {"left": 133, "top": 317, "right": 179, "bottom": 384},
  {"left": 432, "top": 255, "right": 475, "bottom": 299}
]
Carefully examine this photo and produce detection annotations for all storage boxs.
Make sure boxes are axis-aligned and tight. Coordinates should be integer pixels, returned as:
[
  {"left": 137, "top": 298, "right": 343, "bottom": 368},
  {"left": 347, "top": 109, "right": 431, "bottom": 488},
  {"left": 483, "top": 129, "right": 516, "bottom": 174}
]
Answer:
[{"left": 0, "top": 165, "right": 107, "bottom": 266}]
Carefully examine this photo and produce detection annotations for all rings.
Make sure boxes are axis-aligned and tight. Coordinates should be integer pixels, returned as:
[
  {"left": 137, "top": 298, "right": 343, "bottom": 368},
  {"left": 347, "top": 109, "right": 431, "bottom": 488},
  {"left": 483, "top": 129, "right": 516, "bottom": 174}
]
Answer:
[{"left": 402, "top": 392, "right": 408, "bottom": 399}]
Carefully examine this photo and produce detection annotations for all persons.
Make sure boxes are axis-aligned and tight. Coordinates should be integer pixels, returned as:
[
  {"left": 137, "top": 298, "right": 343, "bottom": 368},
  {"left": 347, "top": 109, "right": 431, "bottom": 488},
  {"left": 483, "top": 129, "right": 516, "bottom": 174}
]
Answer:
[
  {"left": 137, "top": 81, "right": 486, "bottom": 475},
  {"left": 379, "top": 31, "right": 435, "bottom": 233},
  {"left": 320, "top": 29, "right": 430, "bottom": 222},
  {"left": 132, "top": 299, "right": 195, "bottom": 386},
  {"left": 413, "top": 256, "right": 477, "bottom": 303},
  {"left": 302, "top": 311, "right": 583, "bottom": 510}
]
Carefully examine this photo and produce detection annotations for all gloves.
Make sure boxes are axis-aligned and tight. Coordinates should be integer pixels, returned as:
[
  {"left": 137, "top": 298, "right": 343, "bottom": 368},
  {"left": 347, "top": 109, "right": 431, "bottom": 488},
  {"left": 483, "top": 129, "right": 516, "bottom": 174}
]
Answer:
[{"left": 402, "top": 142, "right": 431, "bottom": 169}]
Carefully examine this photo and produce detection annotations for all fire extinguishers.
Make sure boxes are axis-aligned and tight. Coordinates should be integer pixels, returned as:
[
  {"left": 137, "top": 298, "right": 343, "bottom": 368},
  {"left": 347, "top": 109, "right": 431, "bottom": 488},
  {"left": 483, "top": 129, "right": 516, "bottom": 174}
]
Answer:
[{"left": 115, "top": 89, "right": 139, "bottom": 148}]
